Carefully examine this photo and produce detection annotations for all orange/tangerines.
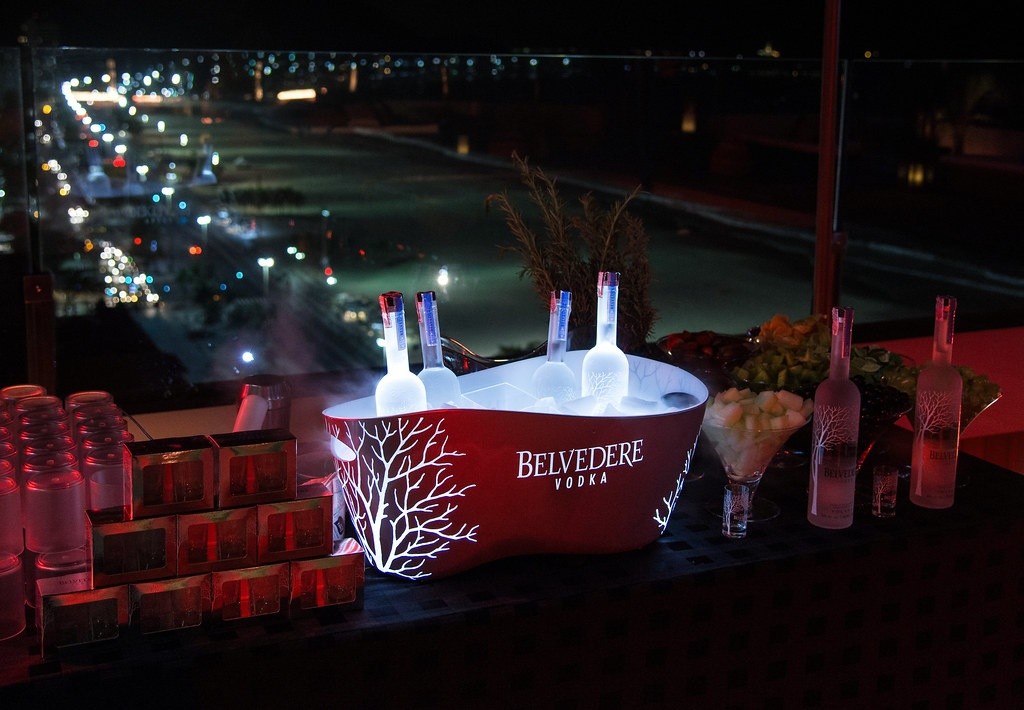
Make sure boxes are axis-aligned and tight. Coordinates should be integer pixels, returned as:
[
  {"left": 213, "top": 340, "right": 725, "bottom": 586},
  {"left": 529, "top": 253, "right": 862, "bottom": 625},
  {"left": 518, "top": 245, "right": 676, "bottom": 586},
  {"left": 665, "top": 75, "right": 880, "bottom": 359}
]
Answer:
[{"left": 756, "top": 312, "right": 820, "bottom": 349}]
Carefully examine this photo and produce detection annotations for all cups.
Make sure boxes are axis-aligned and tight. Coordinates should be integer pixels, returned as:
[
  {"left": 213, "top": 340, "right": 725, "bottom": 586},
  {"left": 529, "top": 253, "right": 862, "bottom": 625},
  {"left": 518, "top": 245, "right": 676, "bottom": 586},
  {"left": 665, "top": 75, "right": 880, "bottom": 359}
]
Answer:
[
  {"left": 871, "top": 464, "right": 899, "bottom": 518},
  {"left": 0, "top": 385, "right": 136, "bottom": 641},
  {"left": 721, "top": 484, "right": 750, "bottom": 538}
]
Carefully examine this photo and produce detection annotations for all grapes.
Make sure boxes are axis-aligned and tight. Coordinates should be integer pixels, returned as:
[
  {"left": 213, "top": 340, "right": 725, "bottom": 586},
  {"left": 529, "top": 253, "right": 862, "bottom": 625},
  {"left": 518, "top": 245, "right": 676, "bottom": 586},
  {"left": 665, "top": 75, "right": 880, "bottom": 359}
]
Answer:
[{"left": 797, "top": 373, "right": 911, "bottom": 419}]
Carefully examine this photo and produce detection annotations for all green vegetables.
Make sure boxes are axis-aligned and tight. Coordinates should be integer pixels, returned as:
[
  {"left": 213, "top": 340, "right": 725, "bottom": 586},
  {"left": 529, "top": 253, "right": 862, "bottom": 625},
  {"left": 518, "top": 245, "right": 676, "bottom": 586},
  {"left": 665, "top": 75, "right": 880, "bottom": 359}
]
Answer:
[{"left": 808, "top": 341, "right": 902, "bottom": 374}]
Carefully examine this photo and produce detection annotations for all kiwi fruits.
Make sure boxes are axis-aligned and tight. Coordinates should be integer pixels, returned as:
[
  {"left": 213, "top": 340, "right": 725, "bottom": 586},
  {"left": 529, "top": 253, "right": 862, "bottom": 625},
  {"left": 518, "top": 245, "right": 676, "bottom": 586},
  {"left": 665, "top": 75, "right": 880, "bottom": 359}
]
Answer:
[{"left": 882, "top": 360, "right": 1002, "bottom": 430}]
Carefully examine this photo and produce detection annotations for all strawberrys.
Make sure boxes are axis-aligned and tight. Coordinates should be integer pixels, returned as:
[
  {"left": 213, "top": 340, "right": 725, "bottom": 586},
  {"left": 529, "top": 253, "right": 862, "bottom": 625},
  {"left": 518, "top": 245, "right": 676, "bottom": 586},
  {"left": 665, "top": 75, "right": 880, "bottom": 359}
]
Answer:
[{"left": 663, "top": 328, "right": 755, "bottom": 393}]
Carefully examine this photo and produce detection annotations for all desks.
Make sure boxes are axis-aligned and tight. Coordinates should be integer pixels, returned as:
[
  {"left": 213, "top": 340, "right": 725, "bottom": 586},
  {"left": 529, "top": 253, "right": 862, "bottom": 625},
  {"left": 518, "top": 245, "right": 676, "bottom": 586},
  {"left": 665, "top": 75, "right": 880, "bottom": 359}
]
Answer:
[
  {"left": 132, "top": 305, "right": 1024, "bottom": 710},
  {"left": 0, "top": 410, "right": 219, "bottom": 710}
]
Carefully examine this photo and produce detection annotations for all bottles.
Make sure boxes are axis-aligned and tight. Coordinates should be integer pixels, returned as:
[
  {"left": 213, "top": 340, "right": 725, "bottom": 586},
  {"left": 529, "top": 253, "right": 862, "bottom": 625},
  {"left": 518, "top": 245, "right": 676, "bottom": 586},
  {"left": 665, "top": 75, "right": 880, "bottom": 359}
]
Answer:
[
  {"left": 375, "top": 290, "right": 428, "bottom": 418},
  {"left": 532, "top": 290, "right": 575, "bottom": 394},
  {"left": 805, "top": 305, "right": 861, "bottom": 530},
  {"left": 415, "top": 291, "right": 461, "bottom": 402},
  {"left": 908, "top": 295, "right": 963, "bottom": 509},
  {"left": 233, "top": 373, "right": 293, "bottom": 431},
  {"left": 581, "top": 271, "right": 630, "bottom": 400}
]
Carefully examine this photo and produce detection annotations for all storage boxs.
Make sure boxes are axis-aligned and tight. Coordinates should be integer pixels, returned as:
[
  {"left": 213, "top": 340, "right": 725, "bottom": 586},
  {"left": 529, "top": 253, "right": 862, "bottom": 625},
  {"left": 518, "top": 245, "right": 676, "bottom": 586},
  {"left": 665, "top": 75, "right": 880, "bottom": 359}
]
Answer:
[{"left": 34, "top": 428, "right": 364, "bottom": 664}]
